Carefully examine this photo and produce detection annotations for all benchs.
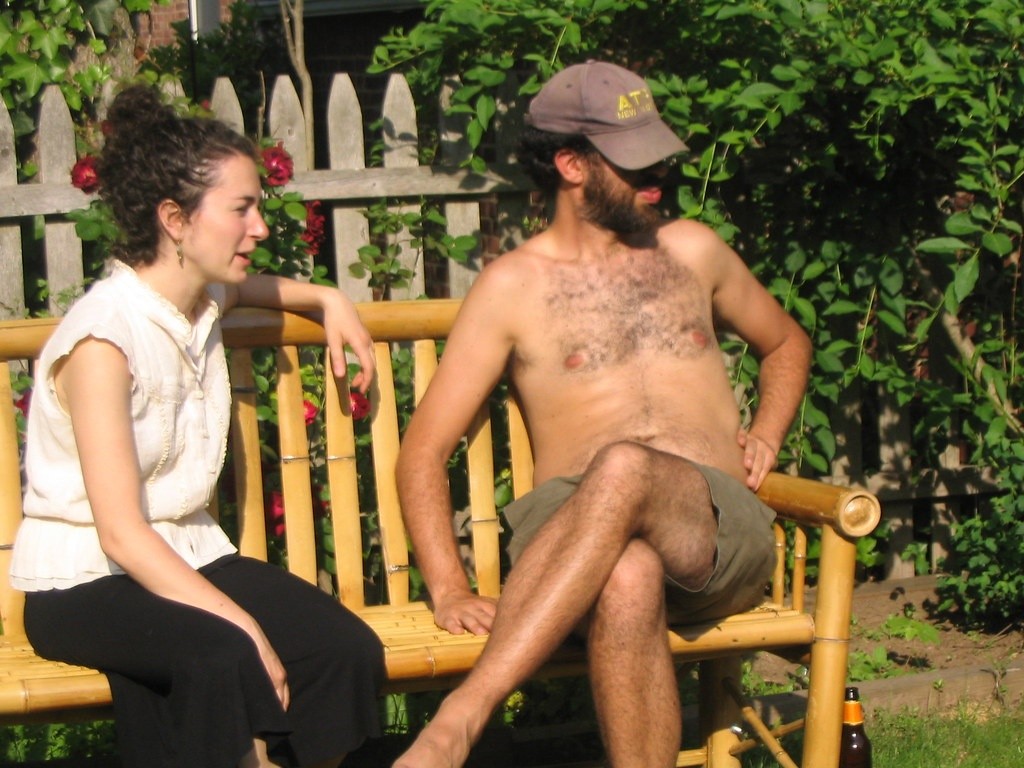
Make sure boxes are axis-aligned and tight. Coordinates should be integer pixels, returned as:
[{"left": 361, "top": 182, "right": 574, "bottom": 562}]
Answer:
[{"left": 0, "top": 298, "right": 881, "bottom": 768}]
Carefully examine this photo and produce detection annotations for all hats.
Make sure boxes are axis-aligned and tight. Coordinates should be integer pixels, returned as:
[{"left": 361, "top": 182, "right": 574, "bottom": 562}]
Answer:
[{"left": 525, "top": 59, "right": 691, "bottom": 170}]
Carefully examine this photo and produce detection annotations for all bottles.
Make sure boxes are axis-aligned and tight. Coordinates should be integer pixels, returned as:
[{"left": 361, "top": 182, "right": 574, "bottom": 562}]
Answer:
[{"left": 839, "top": 687, "right": 874, "bottom": 768}]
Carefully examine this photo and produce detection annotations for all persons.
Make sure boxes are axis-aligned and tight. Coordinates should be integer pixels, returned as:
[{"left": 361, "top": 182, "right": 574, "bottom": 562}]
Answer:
[
  {"left": 390, "top": 58, "right": 813, "bottom": 768},
  {"left": 7, "top": 81, "right": 390, "bottom": 768}
]
